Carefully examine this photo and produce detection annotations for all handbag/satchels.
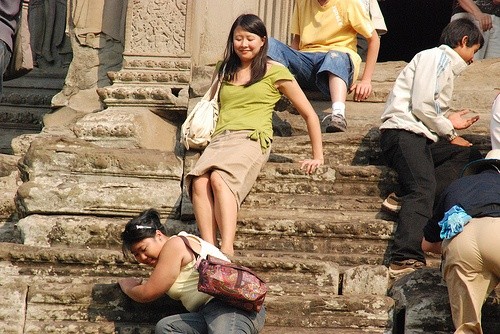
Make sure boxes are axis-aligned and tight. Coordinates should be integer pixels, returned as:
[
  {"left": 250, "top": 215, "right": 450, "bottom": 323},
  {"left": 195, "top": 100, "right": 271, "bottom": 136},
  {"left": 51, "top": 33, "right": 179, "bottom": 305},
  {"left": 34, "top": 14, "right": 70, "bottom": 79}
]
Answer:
[
  {"left": 180, "top": 62, "right": 227, "bottom": 150},
  {"left": 178, "top": 236, "right": 268, "bottom": 313},
  {"left": 2, "top": 0, "right": 34, "bottom": 82}
]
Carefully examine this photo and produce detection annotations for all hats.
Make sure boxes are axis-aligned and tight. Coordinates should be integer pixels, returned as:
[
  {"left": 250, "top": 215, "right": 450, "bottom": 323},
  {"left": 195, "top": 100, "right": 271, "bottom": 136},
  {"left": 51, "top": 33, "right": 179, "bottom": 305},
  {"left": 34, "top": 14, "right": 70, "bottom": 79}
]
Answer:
[{"left": 461, "top": 149, "right": 500, "bottom": 178}]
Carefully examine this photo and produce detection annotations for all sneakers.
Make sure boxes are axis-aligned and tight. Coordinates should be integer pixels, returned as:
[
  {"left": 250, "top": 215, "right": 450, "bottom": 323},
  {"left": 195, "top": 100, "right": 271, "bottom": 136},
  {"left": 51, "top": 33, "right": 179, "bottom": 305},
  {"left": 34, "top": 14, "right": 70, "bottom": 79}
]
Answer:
[
  {"left": 322, "top": 114, "right": 347, "bottom": 132},
  {"left": 382, "top": 193, "right": 409, "bottom": 216},
  {"left": 389, "top": 259, "right": 426, "bottom": 279}
]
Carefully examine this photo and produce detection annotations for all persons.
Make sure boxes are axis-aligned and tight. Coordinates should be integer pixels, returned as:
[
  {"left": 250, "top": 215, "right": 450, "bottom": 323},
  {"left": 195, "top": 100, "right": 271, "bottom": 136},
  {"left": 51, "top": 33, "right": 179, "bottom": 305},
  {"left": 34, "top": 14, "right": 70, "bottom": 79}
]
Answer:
[
  {"left": 421, "top": 150, "right": 500, "bottom": 334},
  {"left": 450, "top": 0, "right": 500, "bottom": 59},
  {"left": 490, "top": 89, "right": 500, "bottom": 149},
  {"left": 119, "top": 208, "right": 268, "bottom": 333},
  {"left": 379, "top": 17, "right": 485, "bottom": 279},
  {"left": 265, "top": 0, "right": 388, "bottom": 132},
  {"left": 0, "top": 0, "right": 21, "bottom": 100},
  {"left": 183, "top": 13, "right": 324, "bottom": 257}
]
